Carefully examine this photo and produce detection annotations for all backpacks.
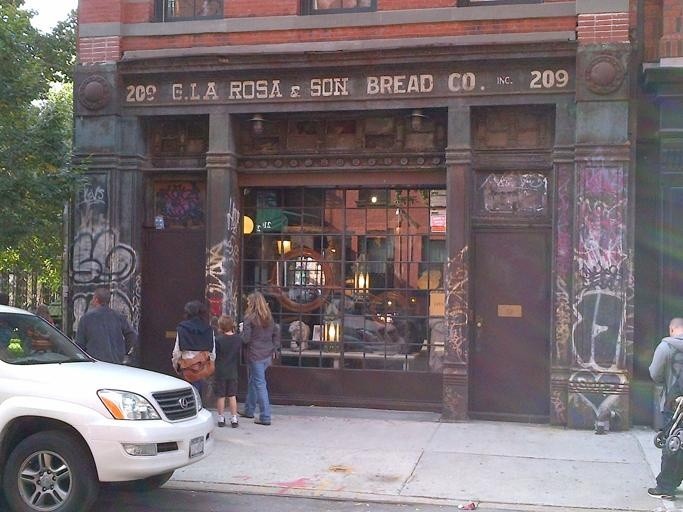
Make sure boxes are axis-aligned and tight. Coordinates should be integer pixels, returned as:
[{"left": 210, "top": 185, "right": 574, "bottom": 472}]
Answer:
[{"left": 662, "top": 362, "right": 683, "bottom": 414}]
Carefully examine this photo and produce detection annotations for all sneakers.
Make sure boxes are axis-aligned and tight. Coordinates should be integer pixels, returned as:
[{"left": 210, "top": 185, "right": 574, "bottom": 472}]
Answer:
[
  {"left": 217, "top": 415, "right": 225, "bottom": 427},
  {"left": 648, "top": 488, "right": 675, "bottom": 500},
  {"left": 254, "top": 420, "right": 270, "bottom": 425},
  {"left": 237, "top": 411, "right": 254, "bottom": 418},
  {"left": 230, "top": 415, "right": 239, "bottom": 428}
]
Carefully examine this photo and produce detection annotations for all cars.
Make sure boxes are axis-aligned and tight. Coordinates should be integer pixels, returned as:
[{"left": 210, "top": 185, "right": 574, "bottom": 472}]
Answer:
[
  {"left": 326, "top": 312, "right": 406, "bottom": 369},
  {"left": 0, "top": 304, "right": 212, "bottom": 512}
]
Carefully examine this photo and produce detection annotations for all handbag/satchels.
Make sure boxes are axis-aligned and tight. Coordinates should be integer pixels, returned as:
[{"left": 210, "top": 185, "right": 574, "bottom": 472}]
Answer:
[{"left": 177, "top": 351, "right": 214, "bottom": 382}]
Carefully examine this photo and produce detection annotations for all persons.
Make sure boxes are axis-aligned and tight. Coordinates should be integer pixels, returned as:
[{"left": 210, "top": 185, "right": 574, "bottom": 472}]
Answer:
[
  {"left": 237, "top": 291, "right": 281, "bottom": 426},
  {"left": 26, "top": 305, "right": 55, "bottom": 353},
  {"left": 647, "top": 315, "right": 683, "bottom": 501},
  {"left": 170, "top": 299, "right": 218, "bottom": 399},
  {"left": 75, "top": 287, "right": 138, "bottom": 364},
  {"left": 215, "top": 314, "right": 244, "bottom": 428}
]
